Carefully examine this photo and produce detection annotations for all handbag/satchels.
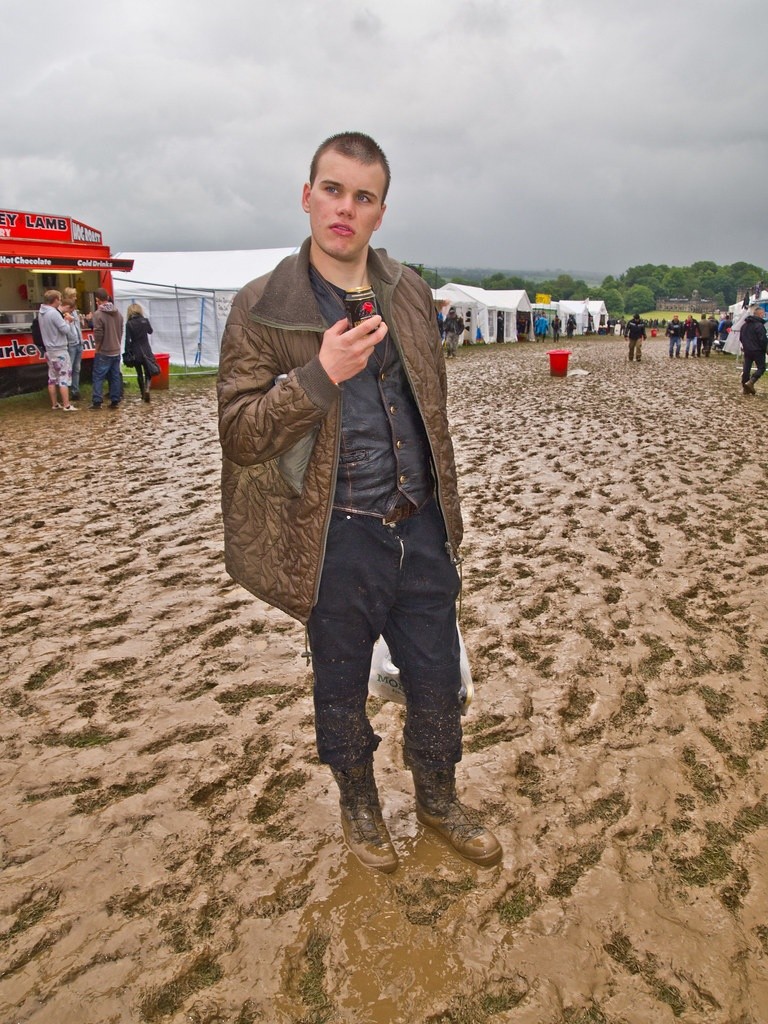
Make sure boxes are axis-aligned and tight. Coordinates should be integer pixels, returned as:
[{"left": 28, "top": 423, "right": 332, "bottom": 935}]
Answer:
[
  {"left": 143, "top": 355, "right": 161, "bottom": 375},
  {"left": 368, "top": 616, "right": 475, "bottom": 716},
  {"left": 123, "top": 349, "right": 136, "bottom": 368}
]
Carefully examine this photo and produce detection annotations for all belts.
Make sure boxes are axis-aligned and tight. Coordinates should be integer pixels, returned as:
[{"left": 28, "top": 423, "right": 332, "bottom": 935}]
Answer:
[{"left": 381, "top": 504, "right": 419, "bottom": 526}]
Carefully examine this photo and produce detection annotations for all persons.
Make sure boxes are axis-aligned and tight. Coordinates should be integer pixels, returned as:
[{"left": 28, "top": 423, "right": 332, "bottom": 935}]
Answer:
[
  {"left": 216, "top": 132, "right": 503, "bottom": 872},
  {"left": 567, "top": 315, "right": 576, "bottom": 338},
  {"left": 534, "top": 314, "right": 549, "bottom": 343},
  {"left": 606, "top": 312, "right": 733, "bottom": 362},
  {"left": 497, "top": 311, "right": 505, "bottom": 343},
  {"left": 740, "top": 308, "right": 768, "bottom": 395},
  {"left": 38, "top": 288, "right": 124, "bottom": 411},
  {"left": 435, "top": 307, "right": 465, "bottom": 358},
  {"left": 551, "top": 316, "right": 562, "bottom": 342},
  {"left": 125, "top": 303, "right": 157, "bottom": 403}
]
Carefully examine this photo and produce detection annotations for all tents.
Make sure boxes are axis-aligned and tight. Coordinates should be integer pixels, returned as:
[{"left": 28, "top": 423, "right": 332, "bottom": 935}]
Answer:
[
  {"left": 428, "top": 281, "right": 608, "bottom": 345},
  {"left": 111, "top": 247, "right": 300, "bottom": 369}
]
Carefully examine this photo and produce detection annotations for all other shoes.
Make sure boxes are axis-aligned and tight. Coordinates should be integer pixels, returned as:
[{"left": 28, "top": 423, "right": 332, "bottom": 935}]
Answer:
[
  {"left": 89, "top": 403, "right": 119, "bottom": 410},
  {"left": 743, "top": 379, "right": 756, "bottom": 394},
  {"left": 144, "top": 391, "right": 150, "bottom": 403},
  {"left": 51, "top": 403, "right": 81, "bottom": 411},
  {"left": 676, "top": 354, "right": 680, "bottom": 358},
  {"left": 705, "top": 350, "right": 708, "bottom": 357},
  {"left": 692, "top": 353, "right": 696, "bottom": 359}
]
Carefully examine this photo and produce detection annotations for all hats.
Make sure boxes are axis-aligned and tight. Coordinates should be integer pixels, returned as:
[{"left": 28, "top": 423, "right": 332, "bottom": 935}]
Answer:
[
  {"left": 634, "top": 314, "right": 640, "bottom": 319},
  {"left": 450, "top": 307, "right": 456, "bottom": 313}
]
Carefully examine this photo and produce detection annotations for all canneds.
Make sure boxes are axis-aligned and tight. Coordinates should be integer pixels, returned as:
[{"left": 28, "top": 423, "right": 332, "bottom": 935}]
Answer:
[{"left": 344, "top": 286, "right": 379, "bottom": 339}]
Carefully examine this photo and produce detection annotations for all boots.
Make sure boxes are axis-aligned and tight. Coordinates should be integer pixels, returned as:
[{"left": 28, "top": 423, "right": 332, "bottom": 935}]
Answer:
[
  {"left": 330, "top": 755, "right": 398, "bottom": 874},
  {"left": 410, "top": 760, "right": 503, "bottom": 866}
]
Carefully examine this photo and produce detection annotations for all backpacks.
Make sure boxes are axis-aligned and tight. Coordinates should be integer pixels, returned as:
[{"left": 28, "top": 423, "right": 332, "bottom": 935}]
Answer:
[{"left": 31, "top": 308, "right": 65, "bottom": 354}]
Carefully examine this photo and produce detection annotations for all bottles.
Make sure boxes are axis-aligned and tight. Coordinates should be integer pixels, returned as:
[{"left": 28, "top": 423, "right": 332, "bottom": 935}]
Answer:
[{"left": 79, "top": 313, "right": 85, "bottom": 328}]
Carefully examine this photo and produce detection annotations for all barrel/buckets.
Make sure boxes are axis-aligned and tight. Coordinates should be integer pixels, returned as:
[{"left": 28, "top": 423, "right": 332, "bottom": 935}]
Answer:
[
  {"left": 546, "top": 350, "right": 572, "bottom": 376},
  {"left": 149, "top": 353, "right": 170, "bottom": 389},
  {"left": 651, "top": 329, "right": 657, "bottom": 337}
]
